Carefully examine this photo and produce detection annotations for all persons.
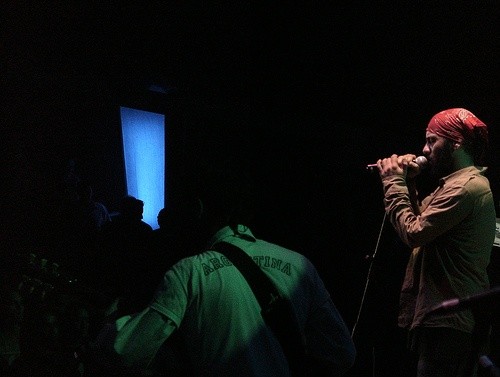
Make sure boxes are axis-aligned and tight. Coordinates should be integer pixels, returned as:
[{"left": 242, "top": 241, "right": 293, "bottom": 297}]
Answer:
[
  {"left": 370, "top": 108, "right": 500, "bottom": 376},
  {"left": 69, "top": 181, "right": 109, "bottom": 231},
  {"left": 110, "top": 184, "right": 360, "bottom": 377}
]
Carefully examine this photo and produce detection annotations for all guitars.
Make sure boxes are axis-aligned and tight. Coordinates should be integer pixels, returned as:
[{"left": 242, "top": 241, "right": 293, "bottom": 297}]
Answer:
[{"left": 13, "top": 252, "right": 156, "bottom": 329}]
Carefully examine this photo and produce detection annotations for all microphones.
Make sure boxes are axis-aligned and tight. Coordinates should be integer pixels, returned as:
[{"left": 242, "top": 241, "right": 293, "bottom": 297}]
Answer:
[
  {"left": 430, "top": 286, "right": 500, "bottom": 315},
  {"left": 366, "top": 156, "right": 428, "bottom": 173}
]
